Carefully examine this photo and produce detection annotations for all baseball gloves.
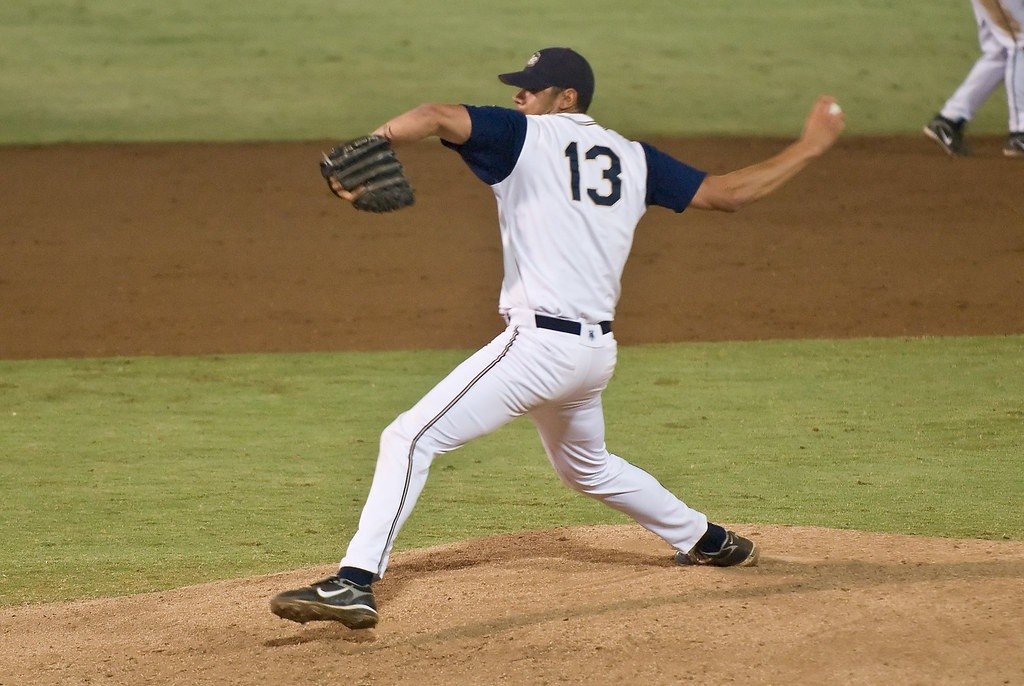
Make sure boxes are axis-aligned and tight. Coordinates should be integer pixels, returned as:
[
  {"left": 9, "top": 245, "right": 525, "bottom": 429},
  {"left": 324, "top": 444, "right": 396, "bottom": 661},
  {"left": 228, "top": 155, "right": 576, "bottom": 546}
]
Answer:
[{"left": 317, "top": 132, "right": 417, "bottom": 214}]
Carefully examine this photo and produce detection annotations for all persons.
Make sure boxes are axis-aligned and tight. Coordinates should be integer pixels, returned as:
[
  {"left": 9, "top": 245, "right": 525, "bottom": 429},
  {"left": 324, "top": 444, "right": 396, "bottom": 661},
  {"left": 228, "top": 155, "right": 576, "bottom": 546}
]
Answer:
[
  {"left": 271, "top": 49, "right": 845, "bottom": 629},
  {"left": 922, "top": 0, "right": 1024, "bottom": 157}
]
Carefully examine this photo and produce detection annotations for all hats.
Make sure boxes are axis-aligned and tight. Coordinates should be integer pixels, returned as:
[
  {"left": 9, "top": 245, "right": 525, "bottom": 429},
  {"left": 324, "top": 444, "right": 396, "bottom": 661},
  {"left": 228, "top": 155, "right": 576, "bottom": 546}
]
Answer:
[{"left": 498, "top": 46, "right": 594, "bottom": 104}]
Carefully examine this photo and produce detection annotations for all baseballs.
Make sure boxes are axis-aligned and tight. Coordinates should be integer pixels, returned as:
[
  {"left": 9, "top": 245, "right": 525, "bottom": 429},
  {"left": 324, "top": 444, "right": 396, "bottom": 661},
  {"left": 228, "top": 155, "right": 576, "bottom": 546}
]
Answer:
[{"left": 826, "top": 102, "right": 842, "bottom": 116}]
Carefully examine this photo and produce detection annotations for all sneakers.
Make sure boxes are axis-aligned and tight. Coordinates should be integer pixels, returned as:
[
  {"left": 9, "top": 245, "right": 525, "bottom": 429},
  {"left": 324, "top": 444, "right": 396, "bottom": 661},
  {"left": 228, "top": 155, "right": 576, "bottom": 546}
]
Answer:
[
  {"left": 673, "top": 522, "right": 760, "bottom": 567},
  {"left": 923, "top": 113, "right": 970, "bottom": 159},
  {"left": 270, "top": 574, "right": 379, "bottom": 630},
  {"left": 1002, "top": 134, "right": 1024, "bottom": 157}
]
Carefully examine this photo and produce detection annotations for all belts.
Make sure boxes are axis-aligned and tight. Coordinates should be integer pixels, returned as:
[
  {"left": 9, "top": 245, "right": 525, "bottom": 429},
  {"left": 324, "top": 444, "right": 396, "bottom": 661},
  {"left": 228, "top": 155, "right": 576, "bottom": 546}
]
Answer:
[{"left": 506, "top": 313, "right": 613, "bottom": 338}]
